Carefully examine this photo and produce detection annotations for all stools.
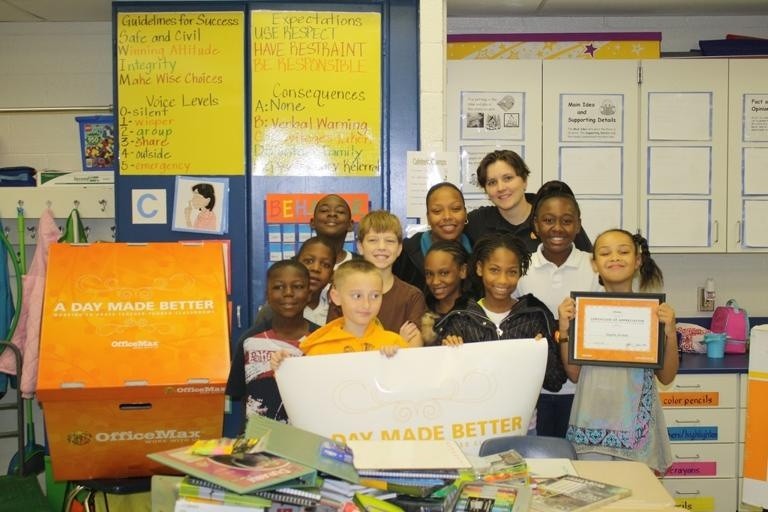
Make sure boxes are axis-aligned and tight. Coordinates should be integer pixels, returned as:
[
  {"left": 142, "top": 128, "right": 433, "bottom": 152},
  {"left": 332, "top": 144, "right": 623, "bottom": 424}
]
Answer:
[{"left": 59, "top": 478, "right": 152, "bottom": 512}]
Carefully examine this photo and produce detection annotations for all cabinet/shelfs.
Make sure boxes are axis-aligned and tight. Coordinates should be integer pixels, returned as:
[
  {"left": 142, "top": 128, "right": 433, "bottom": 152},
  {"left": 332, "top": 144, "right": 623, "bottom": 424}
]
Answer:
[
  {"left": 444, "top": 58, "right": 639, "bottom": 254},
  {"left": 651, "top": 369, "right": 738, "bottom": 512},
  {"left": 640, "top": 57, "right": 768, "bottom": 252},
  {"left": 737, "top": 369, "right": 768, "bottom": 512}
]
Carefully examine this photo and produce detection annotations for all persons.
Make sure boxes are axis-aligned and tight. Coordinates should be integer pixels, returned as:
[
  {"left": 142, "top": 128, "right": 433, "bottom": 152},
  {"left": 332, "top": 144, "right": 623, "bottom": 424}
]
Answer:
[{"left": 557, "top": 230, "right": 680, "bottom": 478}]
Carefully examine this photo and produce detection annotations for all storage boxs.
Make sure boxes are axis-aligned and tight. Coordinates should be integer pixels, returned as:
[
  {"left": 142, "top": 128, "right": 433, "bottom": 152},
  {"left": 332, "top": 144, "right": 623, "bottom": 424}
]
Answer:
[{"left": 34, "top": 242, "right": 231, "bottom": 481}]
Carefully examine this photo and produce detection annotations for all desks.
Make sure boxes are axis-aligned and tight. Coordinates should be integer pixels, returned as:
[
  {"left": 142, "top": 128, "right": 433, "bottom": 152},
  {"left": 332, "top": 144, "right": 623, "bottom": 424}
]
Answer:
[{"left": 156, "top": 438, "right": 679, "bottom": 511}]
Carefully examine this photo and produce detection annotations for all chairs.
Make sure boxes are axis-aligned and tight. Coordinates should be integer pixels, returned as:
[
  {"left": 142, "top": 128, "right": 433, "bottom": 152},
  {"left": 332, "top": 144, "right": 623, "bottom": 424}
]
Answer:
[{"left": 479, "top": 435, "right": 576, "bottom": 460}]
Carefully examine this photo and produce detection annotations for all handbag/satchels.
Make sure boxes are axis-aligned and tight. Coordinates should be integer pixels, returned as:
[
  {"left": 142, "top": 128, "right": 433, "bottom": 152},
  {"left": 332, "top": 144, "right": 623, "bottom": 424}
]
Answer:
[{"left": 710, "top": 300, "right": 748, "bottom": 353}]
